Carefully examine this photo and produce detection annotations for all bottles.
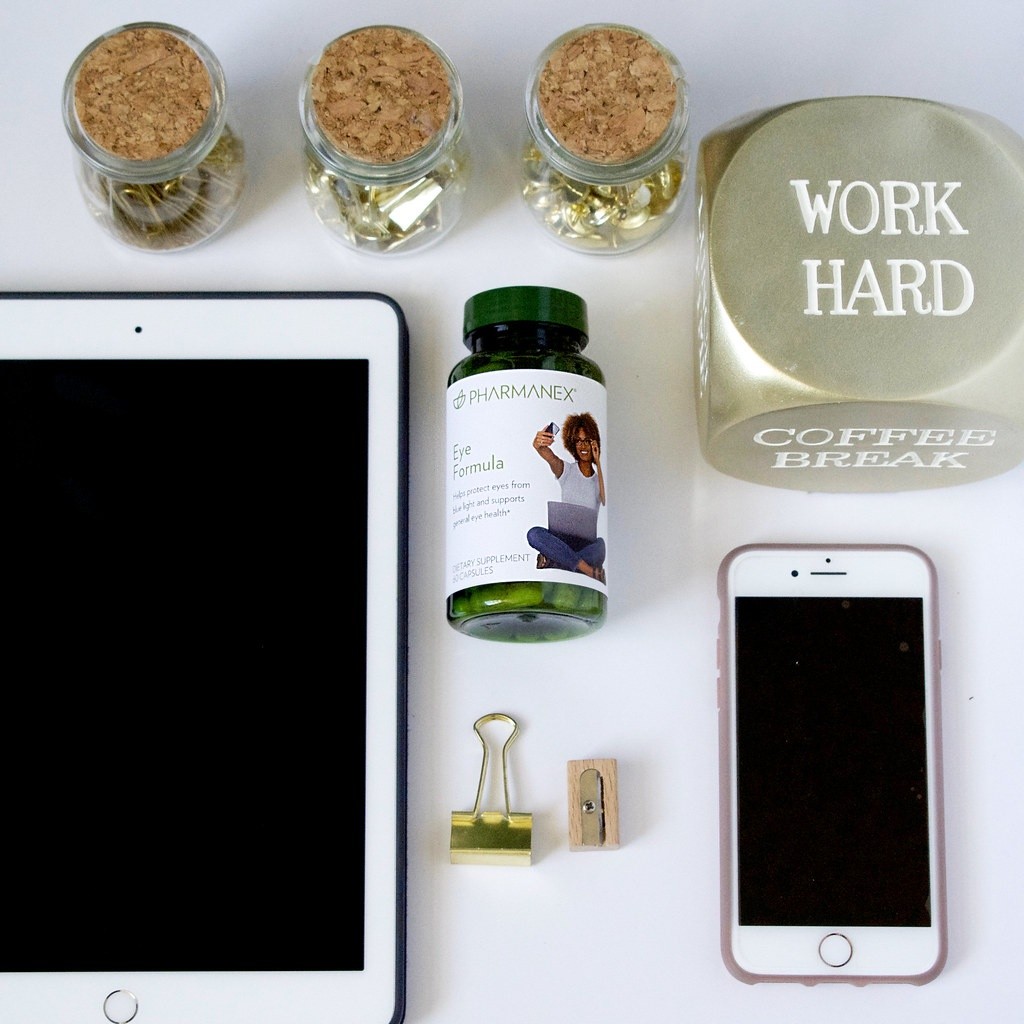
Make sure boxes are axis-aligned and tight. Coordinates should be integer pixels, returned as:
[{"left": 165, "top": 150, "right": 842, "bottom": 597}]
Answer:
[
  {"left": 61, "top": 21, "right": 249, "bottom": 255},
  {"left": 444, "top": 285, "right": 608, "bottom": 644},
  {"left": 514, "top": 22, "right": 691, "bottom": 256},
  {"left": 298, "top": 24, "right": 472, "bottom": 259}
]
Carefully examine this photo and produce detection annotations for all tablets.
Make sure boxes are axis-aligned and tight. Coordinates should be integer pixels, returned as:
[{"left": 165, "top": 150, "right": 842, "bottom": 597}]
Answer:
[{"left": 2, "top": 287, "right": 415, "bottom": 1024}]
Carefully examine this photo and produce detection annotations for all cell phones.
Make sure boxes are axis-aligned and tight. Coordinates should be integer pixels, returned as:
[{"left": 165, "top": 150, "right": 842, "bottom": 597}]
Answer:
[{"left": 718, "top": 544, "right": 949, "bottom": 987}]
